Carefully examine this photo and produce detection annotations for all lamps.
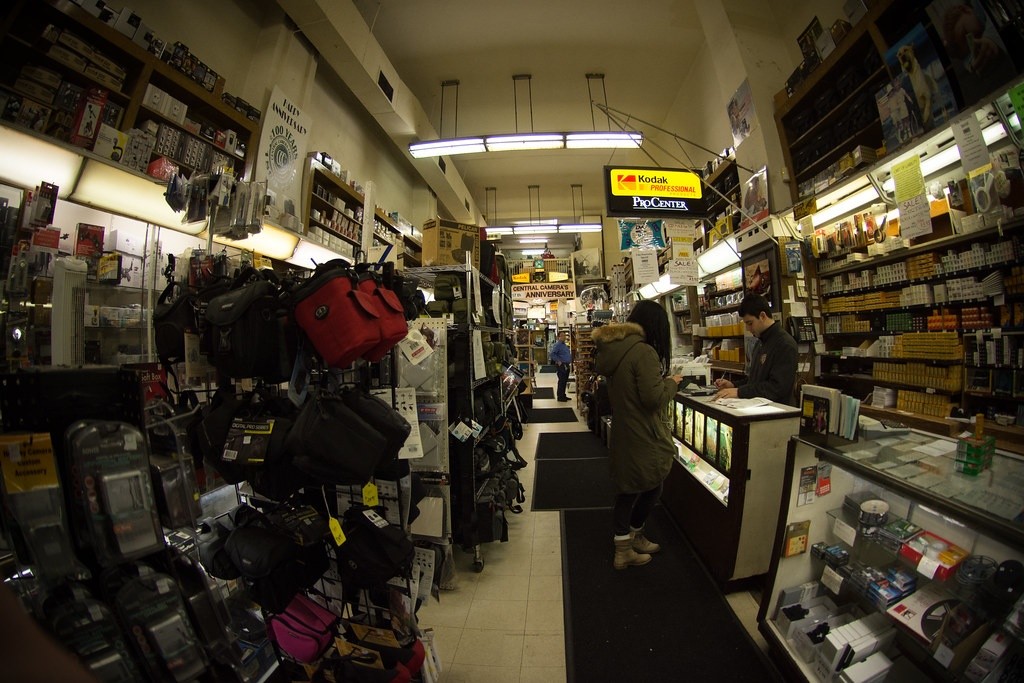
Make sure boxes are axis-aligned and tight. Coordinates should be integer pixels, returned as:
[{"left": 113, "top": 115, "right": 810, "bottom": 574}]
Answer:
[
  {"left": 512, "top": 185, "right": 558, "bottom": 234},
  {"left": 558, "top": 184, "right": 603, "bottom": 234},
  {"left": 637, "top": 232, "right": 742, "bottom": 300},
  {"left": 408, "top": 79, "right": 486, "bottom": 159},
  {"left": 797, "top": 109, "right": 1021, "bottom": 233},
  {"left": 485, "top": 73, "right": 565, "bottom": 152},
  {"left": 565, "top": 73, "right": 644, "bottom": 150},
  {"left": 485, "top": 187, "right": 513, "bottom": 235}
]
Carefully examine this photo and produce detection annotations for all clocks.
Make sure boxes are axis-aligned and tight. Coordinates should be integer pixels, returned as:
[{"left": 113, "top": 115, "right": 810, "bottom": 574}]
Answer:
[{"left": 533, "top": 258, "right": 545, "bottom": 269}]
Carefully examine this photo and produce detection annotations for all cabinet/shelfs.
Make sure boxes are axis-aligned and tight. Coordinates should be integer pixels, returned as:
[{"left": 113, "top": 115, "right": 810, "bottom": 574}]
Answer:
[
  {"left": 300, "top": 157, "right": 364, "bottom": 260},
  {"left": 558, "top": 323, "right": 596, "bottom": 417},
  {"left": 399, "top": 250, "right": 517, "bottom": 572},
  {"left": 773, "top": 0, "right": 966, "bottom": 205},
  {"left": 804, "top": 218, "right": 1024, "bottom": 457},
  {"left": 517, "top": 328, "right": 547, "bottom": 394},
  {"left": 660, "top": 262, "right": 744, "bottom": 374},
  {"left": 28, "top": 276, "right": 160, "bottom": 366},
  {"left": 669, "top": 387, "right": 803, "bottom": 595},
  {"left": 374, "top": 204, "right": 422, "bottom": 268},
  {"left": 704, "top": 152, "right": 741, "bottom": 233},
  {"left": 0, "top": 0, "right": 257, "bottom": 197},
  {"left": 755, "top": 429, "right": 1024, "bottom": 683}
]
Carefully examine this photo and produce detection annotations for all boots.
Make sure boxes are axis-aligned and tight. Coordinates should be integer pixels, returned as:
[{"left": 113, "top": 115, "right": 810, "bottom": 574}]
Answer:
[
  {"left": 613, "top": 536, "right": 652, "bottom": 570},
  {"left": 630, "top": 524, "right": 660, "bottom": 553}
]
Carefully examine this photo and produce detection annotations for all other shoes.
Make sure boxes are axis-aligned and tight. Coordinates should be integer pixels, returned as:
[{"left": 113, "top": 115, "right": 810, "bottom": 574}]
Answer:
[{"left": 557, "top": 396, "right": 571, "bottom": 402}]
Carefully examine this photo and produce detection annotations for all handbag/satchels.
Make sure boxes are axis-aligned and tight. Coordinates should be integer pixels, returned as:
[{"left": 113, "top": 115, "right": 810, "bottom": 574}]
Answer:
[{"left": 153, "top": 253, "right": 427, "bottom": 683}]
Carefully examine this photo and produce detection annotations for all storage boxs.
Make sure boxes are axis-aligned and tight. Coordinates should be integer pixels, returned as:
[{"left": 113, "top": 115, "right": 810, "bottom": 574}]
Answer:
[
  {"left": 772, "top": 490, "right": 1018, "bottom": 683},
  {"left": 112, "top": 251, "right": 142, "bottom": 289},
  {"left": 820, "top": 239, "right": 991, "bottom": 418},
  {"left": 109, "top": 229, "right": 135, "bottom": 254},
  {"left": 422, "top": 215, "right": 481, "bottom": 270},
  {"left": 702, "top": 147, "right": 734, "bottom": 181},
  {"left": 960, "top": 213, "right": 985, "bottom": 233},
  {"left": 707, "top": 320, "right": 745, "bottom": 336},
  {"left": 719, "top": 347, "right": 744, "bottom": 363}
]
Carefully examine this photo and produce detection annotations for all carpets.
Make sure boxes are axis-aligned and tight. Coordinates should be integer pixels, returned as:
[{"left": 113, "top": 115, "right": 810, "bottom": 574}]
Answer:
[{"left": 527, "top": 365, "right": 784, "bottom": 683}]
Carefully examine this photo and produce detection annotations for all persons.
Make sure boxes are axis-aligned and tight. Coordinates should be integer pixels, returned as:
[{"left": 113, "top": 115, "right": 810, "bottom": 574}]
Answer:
[
  {"left": 885, "top": 80, "right": 913, "bottom": 143},
  {"left": 549, "top": 330, "right": 572, "bottom": 402},
  {"left": 939, "top": 0, "right": 1009, "bottom": 72},
  {"left": 590, "top": 300, "right": 683, "bottom": 569},
  {"left": 711, "top": 293, "right": 798, "bottom": 405}
]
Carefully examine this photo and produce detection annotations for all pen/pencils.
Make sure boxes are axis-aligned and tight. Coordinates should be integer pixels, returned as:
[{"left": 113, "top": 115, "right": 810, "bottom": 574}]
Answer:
[{"left": 720, "top": 371, "right": 726, "bottom": 381}]
